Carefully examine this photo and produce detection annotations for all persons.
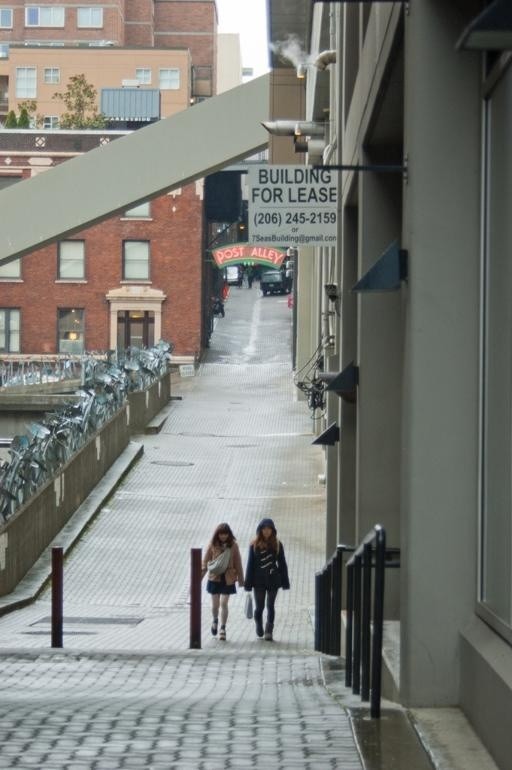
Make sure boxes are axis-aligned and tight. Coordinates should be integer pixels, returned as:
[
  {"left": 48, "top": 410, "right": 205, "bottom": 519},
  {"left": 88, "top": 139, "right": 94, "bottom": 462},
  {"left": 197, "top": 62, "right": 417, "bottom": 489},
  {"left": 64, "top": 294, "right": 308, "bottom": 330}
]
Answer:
[
  {"left": 243, "top": 518, "right": 290, "bottom": 642},
  {"left": 237, "top": 264, "right": 257, "bottom": 289},
  {"left": 200, "top": 522, "right": 246, "bottom": 641}
]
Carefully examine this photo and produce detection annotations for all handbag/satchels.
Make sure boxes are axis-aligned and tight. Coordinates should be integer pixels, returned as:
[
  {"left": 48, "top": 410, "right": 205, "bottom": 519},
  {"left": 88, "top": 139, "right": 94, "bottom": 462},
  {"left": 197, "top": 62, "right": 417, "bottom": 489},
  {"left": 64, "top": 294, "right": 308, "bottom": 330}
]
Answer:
[
  {"left": 207, "top": 548, "right": 231, "bottom": 576},
  {"left": 244, "top": 595, "right": 253, "bottom": 619},
  {"left": 264, "top": 570, "right": 283, "bottom": 589}
]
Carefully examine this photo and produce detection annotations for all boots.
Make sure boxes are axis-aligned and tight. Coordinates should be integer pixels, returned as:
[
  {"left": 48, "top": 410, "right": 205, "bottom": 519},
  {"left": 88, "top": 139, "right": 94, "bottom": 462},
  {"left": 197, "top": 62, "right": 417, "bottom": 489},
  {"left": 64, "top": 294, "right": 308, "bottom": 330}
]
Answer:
[{"left": 255, "top": 619, "right": 274, "bottom": 640}]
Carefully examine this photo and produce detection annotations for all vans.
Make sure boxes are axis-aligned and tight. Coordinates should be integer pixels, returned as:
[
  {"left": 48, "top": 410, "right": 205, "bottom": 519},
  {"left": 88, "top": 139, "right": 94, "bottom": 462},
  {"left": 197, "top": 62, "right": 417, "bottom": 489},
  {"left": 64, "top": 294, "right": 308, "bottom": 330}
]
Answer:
[{"left": 223, "top": 266, "right": 243, "bottom": 284}]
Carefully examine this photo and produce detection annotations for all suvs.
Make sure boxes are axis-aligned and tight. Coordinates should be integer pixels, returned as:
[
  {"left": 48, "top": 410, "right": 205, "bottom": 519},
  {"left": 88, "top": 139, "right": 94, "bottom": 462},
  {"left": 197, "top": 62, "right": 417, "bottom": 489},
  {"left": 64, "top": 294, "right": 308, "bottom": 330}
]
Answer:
[{"left": 260, "top": 271, "right": 290, "bottom": 295}]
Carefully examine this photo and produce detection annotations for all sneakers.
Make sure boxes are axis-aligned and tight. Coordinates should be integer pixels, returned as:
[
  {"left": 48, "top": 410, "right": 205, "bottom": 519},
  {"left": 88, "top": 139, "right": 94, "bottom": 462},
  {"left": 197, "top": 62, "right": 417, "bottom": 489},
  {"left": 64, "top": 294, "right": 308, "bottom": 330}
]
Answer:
[{"left": 211, "top": 621, "right": 226, "bottom": 640}]
